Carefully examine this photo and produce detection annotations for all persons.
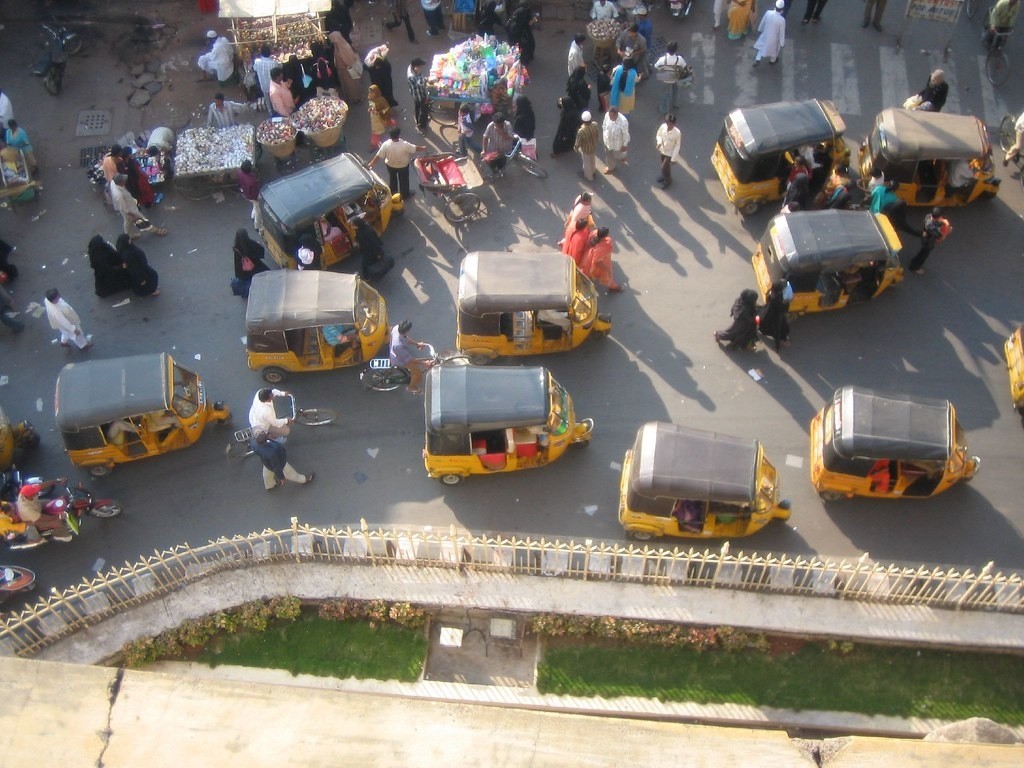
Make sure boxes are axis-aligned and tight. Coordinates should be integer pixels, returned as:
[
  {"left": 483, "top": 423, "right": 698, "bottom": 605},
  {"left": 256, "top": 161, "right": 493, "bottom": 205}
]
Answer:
[
  {"left": 0, "top": 237, "right": 25, "bottom": 334},
  {"left": 321, "top": 325, "right": 361, "bottom": 349},
  {"left": 713, "top": 0, "right": 793, "bottom": 68},
  {"left": 801, "top": 0, "right": 827, "bottom": 26},
  {"left": 776, "top": 69, "right": 980, "bottom": 276},
  {"left": 672, "top": 499, "right": 700, "bottom": 534},
  {"left": 1003, "top": 111, "right": 1024, "bottom": 168},
  {"left": 537, "top": 309, "right": 572, "bottom": 333},
  {"left": 100, "top": 409, "right": 182, "bottom": 440},
  {"left": 713, "top": 278, "right": 791, "bottom": 351},
  {"left": 389, "top": 319, "right": 432, "bottom": 393},
  {"left": 557, "top": 192, "right": 626, "bottom": 293},
  {"left": 0, "top": 477, "right": 73, "bottom": 544},
  {"left": 195, "top": 0, "right": 538, "bottom": 283},
  {"left": 0, "top": 91, "right": 41, "bottom": 176},
  {"left": 43, "top": 288, "right": 94, "bottom": 352},
  {"left": 550, "top": 0, "right": 688, "bottom": 191},
  {"left": 980, "top": 0, "right": 1021, "bottom": 54},
  {"left": 88, "top": 127, "right": 176, "bottom": 298},
  {"left": 248, "top": 387, "right": 315, "bottom": 492},
  {"left": 861, "top": 0, "right": 888, "bottom": 32}
]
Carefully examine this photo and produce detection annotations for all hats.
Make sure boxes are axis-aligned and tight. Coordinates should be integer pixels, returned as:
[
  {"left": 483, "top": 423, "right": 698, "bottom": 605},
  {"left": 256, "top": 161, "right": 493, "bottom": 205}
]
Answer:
[
  {"left": 207, "top": 30, "right": 218, "bottom": 38},
  {"left": 581, "top": 111, "right": 592, "bottom": 122},
  {"left": 412, "top": 57, "right": 426, "bottom": 67},
  {"left": 635, "top": 8, "right": 647, "bottom": 15},
  {"left": 776, "top": 0, "right": 785, "bottom": 9},
  {"left": 21, "top": 483, "right": 41, "bottom": 498}
]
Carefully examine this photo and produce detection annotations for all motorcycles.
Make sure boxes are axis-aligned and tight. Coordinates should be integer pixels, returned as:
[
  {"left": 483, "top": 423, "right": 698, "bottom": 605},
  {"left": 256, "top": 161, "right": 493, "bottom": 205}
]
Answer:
[
  {"left": 423, "top": 364, "right": 594, "bottom": 486},
  {"left": 617, "top": 421, "right": 792, "bottom": 544},
  {"left": 1005, "top": 323, "right": 1024, "bottom": 412},
  {"left": 8, "top": 473, "right": 125, "bottom": 548},
  {"left": 54, "top": 351, "right": 234, "bottom": 478},
  {"left": 858, "top": 107, "right": 1002, "bottom": 217},
  {"left": 809, "top": 386, "right": 981, "bottom": 503},
  {"left": 257, "top": 152, "right": 403, "bottom": 270},
  {"left": 711, "top": 97, "right": 850, "bottom": 216},
  {"left": 751, "top": 209, "right": 903, "bottom": 323},
  {"left": 668, "top": 0, "right": 691, "bottom": 21},
  {"left": 0, "top": 403, "right": 56, "bottom": 504},
  {"left": 244, "top": 269, "right": 394, "bottom": 384},
  {"left": 456, "top": 251, "right": 612, "bottom": 365}
]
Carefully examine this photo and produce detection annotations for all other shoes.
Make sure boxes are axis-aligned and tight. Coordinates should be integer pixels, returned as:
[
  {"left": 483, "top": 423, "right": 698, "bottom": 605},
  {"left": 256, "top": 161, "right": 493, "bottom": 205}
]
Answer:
[
  {"left": 60, "top": 342, "right": 70, "bottom": 347},
  {"left": 302, "top": 472, "right": 313, "bottom": 485},
  {"left": 912, "top": 269, "right": 924, "bottom": 274},
  {"left": 54, "top": 535, "right": 73, "bottom": 542},
  {"left": 80, "top": 341, "right": 93, "bottom": 350},
  {"left": 427, "top": 30, "right": 440, "bottom": 38},
  {"left": 369, "top": 146, "right": 377, "bottom": 154},
  {"left": 132, "top": 234, "right": 142, "bottom": 240},
  {"left": 752, "top": 17, "right": 884, "bottom": 69},
  {"left": 1003, "top": 156, "right": 1008, "bottom": 166},
  {"left": 610, "top": 285, "right": 624, "bottom": 291},
  {"left": 194, "top": 78, "right": 207, "bottom": 82},
  {"left": 155, "top": 229, "right": 167, "bottom": 235},
  {"left": 578, "top": 167, "right": 670, "bottom": 191},
  {"left": 406, "top": 386, "right": 424, "bottom": 396},
  {"left": 714, "top": 331, "right": 722, "bottom": 345}
]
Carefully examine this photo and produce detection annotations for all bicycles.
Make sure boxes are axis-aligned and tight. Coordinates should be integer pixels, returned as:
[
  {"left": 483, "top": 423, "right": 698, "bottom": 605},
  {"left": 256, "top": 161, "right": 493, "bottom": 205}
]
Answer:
[
  {"left": 962, "top": 0, "right": 1014, "bottom": 85},
  {"left": 29, "top": 10, "right": 175, "bottom": 97},
  {"left": 359, "top": 341, "right": 471, "bottom": 393},
  {"left": 998, "top": 114, "right": 1024, "bottom": 192},
  {"left": 225, "top": 392, "right": 337, "bottom": 460}
]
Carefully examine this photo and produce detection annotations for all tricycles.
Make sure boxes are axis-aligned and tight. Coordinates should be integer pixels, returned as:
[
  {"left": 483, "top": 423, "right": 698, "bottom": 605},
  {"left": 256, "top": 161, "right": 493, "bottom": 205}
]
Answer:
[{"left": 409, "top": 138, "right": 548, "bottom": 223}]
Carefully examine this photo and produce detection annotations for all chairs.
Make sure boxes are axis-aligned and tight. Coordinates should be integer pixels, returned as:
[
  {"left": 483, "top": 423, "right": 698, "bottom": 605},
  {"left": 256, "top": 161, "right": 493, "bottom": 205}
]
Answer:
[
  {"left": 513, "top": 426, "right": 537, "bottom": 457},
  {"left": 534, "top": 311, "right": 562, "bottom": 339}
]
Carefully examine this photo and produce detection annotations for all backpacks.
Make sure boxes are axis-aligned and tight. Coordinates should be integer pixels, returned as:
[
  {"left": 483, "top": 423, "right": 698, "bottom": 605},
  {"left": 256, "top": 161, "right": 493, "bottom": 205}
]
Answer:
[{"left": 656, "top": 54, "right": 682, "bottom": 82}]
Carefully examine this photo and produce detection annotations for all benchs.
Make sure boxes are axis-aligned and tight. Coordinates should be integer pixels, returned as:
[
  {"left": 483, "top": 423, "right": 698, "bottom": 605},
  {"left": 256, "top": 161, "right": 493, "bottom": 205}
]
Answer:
[
  {"left": 434, "top": 155, "right": 465, "bottom": 188},
  {"left": 471, "top": 438, "right": 487, "bottom": 456},
  {"left": 320, "top": 218, "right": 349, "bottom": 255},
  {"left": 284, "top": 329, "right": 304, "bottom": 357}
]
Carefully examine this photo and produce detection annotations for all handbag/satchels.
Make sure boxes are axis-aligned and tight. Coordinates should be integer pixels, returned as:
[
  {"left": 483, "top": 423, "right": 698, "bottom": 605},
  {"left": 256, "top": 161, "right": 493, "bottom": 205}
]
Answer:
[
  {"left": 242, "top": 257, "right": 255, "bottom": 272},
  {"left": 133, "top": 217, "right": 152, "bottom": 231},
  {"left": 301, "top": 64, "right": 313, "bottom": 88},
  {"left": 347, "top": 53, "right": 364, "bottom": 80}
]
